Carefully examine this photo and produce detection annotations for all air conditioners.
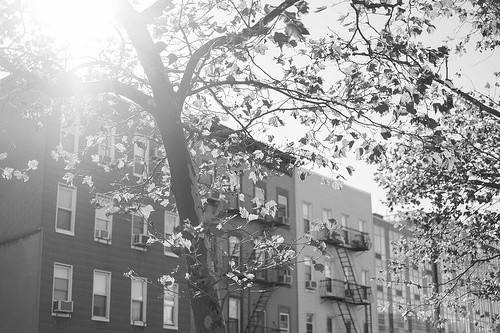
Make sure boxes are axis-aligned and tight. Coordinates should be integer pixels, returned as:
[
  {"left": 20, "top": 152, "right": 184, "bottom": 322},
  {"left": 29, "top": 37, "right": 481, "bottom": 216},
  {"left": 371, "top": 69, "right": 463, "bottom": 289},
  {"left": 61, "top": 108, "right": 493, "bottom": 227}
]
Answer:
[
  {"left": 96, "top": 229, "right": 109, "bottom": 239},
  {"left": 209, "top": 191, "right": 221, "bottom": 201},
  {"left": 53, "top": 300, "right": 74, "bottom": 312},
  {"left": 279, "top": 275, "right": 292, "bottom": 285},
  {"left": 306, "top": 280, "right": 317, "bottom": 289},
  {"left": 279, "top": 216, "right": 290, "bottom": 224}
]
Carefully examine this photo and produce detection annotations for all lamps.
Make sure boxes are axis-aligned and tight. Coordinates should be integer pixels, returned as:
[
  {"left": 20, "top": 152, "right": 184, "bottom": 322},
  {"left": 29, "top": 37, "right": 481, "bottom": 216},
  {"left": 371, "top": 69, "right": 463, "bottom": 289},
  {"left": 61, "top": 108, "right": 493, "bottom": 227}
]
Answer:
[{"left": 133, "top": 234, "right": 151, "bottom": 246}]
